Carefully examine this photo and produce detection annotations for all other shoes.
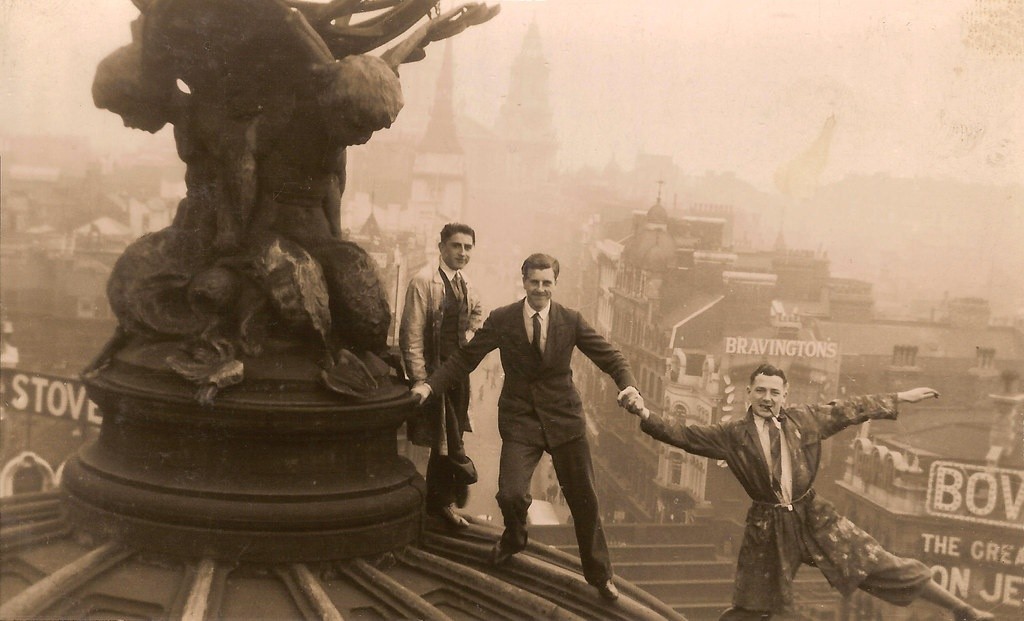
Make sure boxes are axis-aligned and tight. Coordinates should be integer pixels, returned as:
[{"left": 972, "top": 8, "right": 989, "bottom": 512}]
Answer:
[
  {"left": 491, "top": 542, "right": 513, "bottom": 567},
  {"left": 426, "top": 501, "right": 470, "bottom": 530},
  {"left": 956, "top": 604, "right": 994, "bottom": 621},
  {"left": 585, "top": 577, "right": 619, "bottom": 600}
]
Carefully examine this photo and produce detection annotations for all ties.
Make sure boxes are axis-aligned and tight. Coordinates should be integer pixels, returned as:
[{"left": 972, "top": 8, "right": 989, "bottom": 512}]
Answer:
[
  {"left": 764, "top": 416, "right": 784, "bottom": 500},
  {"left": 531, "top": 313, "right": 541, "bottom": 355},
  {"left": 455, "top": 271, "right": 463, "bottom": 296}
]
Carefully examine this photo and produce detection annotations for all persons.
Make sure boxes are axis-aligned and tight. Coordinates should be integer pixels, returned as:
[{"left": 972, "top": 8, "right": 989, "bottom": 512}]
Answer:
[
  {"left": 616, "top": 364, "right": 996, "bottom": 621},
  {"left": 411, "top": 255, "right": 644, "bottom": 600},
  {"left": 399, "top": 224, "right": 485, "bottom": 527}
]
